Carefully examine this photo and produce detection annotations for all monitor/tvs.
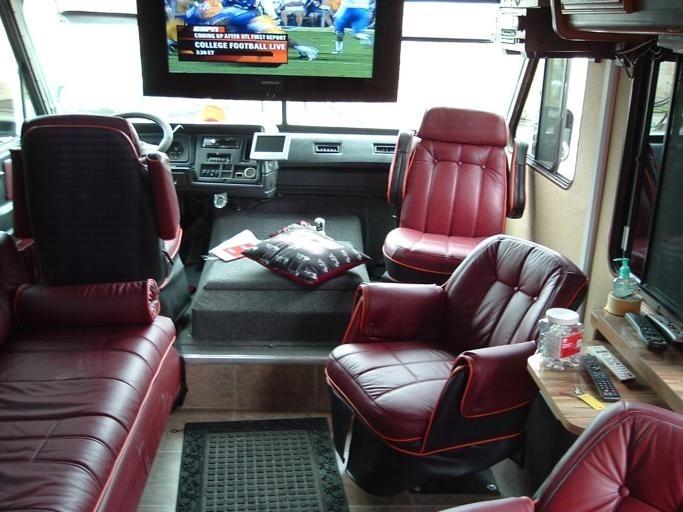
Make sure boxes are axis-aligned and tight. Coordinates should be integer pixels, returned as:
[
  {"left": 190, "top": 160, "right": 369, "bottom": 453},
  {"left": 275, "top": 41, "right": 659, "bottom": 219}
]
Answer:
[{"left": 135, "top": 1, "right": 404, "bottom": 103}]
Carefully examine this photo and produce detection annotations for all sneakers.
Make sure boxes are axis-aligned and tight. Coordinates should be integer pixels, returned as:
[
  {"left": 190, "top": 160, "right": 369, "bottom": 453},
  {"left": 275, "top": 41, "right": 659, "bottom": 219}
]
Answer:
[
  {"left": 331, "top": 49, "right": 343, "bottom": 55},
  {"left": 328, "top": 25, "right": 334, "bottom": 30},
  {"left": 294, "top": 44, "right": 319, "bottom": 61}
]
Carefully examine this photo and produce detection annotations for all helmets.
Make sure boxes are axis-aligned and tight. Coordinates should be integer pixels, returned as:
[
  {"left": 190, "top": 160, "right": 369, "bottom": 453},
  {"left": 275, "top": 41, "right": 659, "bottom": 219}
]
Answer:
[{"left": 201, "top": 0, "right": 224, "bottom": 20}]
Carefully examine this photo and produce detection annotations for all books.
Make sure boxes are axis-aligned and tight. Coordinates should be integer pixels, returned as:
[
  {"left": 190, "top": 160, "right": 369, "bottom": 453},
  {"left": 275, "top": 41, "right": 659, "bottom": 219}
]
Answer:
[{"left": 208, "top": 228, "right": 264, "bottom": 262}]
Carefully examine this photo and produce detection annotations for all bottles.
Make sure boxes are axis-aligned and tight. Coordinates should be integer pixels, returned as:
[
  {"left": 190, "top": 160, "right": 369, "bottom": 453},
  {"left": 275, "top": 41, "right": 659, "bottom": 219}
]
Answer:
[{"left": 537, "top": 306, "right": 585, "bottom": 371}]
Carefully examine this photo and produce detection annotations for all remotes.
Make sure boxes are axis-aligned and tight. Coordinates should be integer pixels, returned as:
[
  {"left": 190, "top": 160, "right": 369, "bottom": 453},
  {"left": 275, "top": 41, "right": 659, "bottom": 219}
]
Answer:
[
  {"left": 582, "top": 355, "right": 621, "bottom": 402},
  {"left": 588, "top": 345, "right": 636, "bottom": 382},
  {"left": 625, "top": 312, "right": 668, "bottom": 351},
  {"left": 646, "top": 313, "right": 683, "bottom": 342}
]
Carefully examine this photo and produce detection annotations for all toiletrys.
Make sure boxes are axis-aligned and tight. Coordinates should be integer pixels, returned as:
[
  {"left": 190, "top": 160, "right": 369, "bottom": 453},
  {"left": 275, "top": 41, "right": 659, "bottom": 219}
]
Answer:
[{"left": 611, "top": 257, "right": 639, "bottom": 298}]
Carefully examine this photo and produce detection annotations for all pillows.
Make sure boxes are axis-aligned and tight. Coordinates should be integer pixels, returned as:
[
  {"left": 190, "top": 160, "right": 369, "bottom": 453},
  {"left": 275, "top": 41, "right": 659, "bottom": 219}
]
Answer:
[{"left": 240, "top": 224, "right": 373, "bottom": 289}]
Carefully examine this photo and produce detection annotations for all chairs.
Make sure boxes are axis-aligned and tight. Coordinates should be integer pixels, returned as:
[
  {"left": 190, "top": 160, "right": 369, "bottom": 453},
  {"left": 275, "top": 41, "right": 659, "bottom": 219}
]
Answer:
[{"left": 8, "top": 113, "right": 193, "bottom": 322}]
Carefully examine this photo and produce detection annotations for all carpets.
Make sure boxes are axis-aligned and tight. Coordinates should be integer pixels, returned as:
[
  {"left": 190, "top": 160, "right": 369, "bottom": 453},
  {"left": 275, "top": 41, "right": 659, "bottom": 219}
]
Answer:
[{"left": 174, "top": 416, "right": 351, "bottom": 512}]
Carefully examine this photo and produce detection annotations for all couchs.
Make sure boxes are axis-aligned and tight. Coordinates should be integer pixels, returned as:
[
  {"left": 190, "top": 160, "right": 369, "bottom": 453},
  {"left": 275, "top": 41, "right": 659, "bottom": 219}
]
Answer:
[{"left": 0, "top": 230, "right": 182, "bottom": 512}]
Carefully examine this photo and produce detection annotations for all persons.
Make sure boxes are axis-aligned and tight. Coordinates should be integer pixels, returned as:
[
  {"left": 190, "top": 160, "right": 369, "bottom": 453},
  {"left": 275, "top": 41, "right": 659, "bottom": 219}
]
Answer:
[{"left": 164, "top": 0, "right": 375, "bottom": 63}]
